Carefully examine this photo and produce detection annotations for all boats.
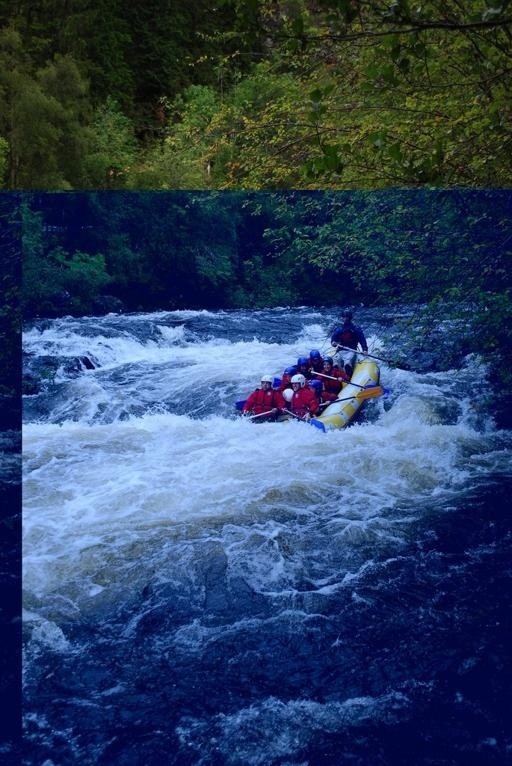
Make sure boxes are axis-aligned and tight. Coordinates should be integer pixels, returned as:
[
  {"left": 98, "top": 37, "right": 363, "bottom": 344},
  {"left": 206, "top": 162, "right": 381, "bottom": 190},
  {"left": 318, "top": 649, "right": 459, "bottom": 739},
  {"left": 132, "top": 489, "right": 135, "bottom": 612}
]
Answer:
[{"left": 251, "top": 347, "right": 384, "bottom": 434}]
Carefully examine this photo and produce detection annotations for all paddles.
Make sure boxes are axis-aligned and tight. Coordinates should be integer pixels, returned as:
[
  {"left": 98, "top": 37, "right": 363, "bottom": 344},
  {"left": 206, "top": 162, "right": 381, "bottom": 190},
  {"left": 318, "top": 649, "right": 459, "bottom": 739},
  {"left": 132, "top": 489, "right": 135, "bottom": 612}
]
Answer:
[
  {"left": 337, "top": 343, "right": 411, "bottom": 370},
  {"left": 319, "top": 385, "right": 384, "bottom": 406},
  {"left": 311, "top": 370, "right": 389, "bottom": 399},
  {"left": 282, "top": 408, "right": 326, "bottom": 434}
]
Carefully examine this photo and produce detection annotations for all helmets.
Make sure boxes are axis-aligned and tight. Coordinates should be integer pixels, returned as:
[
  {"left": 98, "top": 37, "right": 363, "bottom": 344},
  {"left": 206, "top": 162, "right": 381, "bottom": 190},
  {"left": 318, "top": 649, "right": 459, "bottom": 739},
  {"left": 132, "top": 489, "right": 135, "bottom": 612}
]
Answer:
[
  {"left": 261, "top": 350, "right": 333, "bottom": 389},
  {"left": 341, "top": 310, "right": 353, "bottom": 317}
]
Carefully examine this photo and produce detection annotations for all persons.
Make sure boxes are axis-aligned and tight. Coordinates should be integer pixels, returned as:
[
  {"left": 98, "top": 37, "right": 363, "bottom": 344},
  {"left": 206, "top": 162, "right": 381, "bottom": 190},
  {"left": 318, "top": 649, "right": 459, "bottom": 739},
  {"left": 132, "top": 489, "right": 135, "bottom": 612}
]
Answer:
[
  {"left": 278, "top": 349, "right": 351, "bottom": 421},
  {"left": 242, "top": 375, "right": 286, "bottom": 423},
  {"left": 330, "top": 309, "right": 368, "bottom": 377}
]
